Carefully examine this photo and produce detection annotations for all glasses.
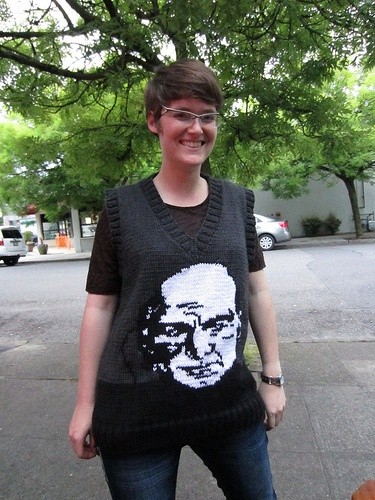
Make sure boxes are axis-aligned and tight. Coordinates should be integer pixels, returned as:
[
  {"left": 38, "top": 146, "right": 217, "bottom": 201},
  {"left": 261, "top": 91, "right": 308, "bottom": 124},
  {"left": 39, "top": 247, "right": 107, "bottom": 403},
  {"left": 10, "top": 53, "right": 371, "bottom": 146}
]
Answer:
[{"left": 159, "top": 104, "right": 220, "bottom": 125}]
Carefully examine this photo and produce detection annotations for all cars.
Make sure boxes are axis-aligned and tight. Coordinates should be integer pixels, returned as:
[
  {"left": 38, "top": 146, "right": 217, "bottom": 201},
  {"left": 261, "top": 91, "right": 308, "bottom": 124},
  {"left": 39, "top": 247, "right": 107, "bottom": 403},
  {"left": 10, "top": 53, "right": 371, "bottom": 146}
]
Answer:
[
  {"left": 253, "top": 212, "right": 292, "bottom": 251},
  {"left": 82, "top": 225, "right": 97, "bottom": 237}
]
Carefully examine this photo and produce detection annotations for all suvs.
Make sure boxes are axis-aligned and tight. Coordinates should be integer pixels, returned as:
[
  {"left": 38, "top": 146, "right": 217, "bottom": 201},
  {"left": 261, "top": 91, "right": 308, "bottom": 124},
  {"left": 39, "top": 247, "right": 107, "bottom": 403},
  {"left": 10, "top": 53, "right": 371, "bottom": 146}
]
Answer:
[{"left": 0, "top": 225, "right": 28, "bottom": 266}]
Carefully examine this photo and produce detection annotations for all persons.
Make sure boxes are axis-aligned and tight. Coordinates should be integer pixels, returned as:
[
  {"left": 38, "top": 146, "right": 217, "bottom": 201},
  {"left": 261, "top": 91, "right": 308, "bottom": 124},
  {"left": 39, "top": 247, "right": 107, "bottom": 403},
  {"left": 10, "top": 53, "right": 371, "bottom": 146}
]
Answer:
[{"left": 68, "top": 59, "right": 320, "bottom": 499}]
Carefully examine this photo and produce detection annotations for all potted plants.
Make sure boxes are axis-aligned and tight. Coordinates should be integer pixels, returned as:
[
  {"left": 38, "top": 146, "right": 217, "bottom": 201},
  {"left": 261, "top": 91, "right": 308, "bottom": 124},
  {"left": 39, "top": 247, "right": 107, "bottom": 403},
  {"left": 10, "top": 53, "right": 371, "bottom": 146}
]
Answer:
[
  {"left": 304, "top": 213, "right": 341, "bottom": 236},
  {"left": 23, "top": 231, "right": 35, "bottom": 252},
  {"left": 38, "top": 238, "right": 48, "bottom": 255}
]
